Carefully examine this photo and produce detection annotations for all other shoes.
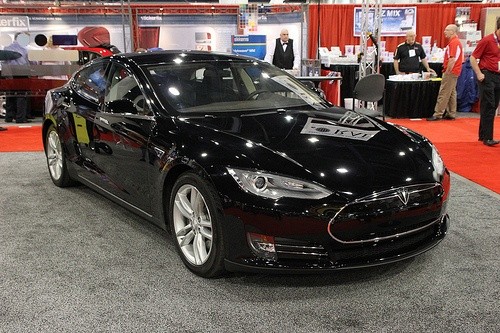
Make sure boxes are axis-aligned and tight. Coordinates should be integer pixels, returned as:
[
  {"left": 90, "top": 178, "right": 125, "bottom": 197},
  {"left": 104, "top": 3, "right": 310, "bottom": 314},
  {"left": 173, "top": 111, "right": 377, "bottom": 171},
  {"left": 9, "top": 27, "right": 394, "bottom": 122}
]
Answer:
[
  {"left": 484, "top": 139, "right": 499, "bottom": 145},
  {"left": 444, "top": 114, "right": 454, "bottom": 119},
  {"left": 426, "top": 117, "right": 442, "bottom": 121},
  {"left": 478, "top": 136, "right": 484, "bottom": 141}
]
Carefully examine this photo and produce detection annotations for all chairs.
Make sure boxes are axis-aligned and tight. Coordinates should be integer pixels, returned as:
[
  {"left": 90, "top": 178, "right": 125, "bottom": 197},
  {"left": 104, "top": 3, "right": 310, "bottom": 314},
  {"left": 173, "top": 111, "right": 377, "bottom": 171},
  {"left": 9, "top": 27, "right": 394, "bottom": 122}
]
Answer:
[
  {"left": 142, "top": 75, "right": 183, "bottom": 115},
  {"left": 197, "top": 69, "right": 240, "bottom": 106},
  {"left": 352, "top": 74, "right": 387, "bottom": 120}
]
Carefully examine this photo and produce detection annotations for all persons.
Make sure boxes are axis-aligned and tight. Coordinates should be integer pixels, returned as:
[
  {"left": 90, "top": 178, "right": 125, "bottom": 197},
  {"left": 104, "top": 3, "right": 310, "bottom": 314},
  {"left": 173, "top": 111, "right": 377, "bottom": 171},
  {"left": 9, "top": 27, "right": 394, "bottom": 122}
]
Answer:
[
  {"left": 426, "top": 24, "right": 463, "bottom": 121},
  {"left": 393, "top": 30, "right": 436, "bottom": 77},
  {"left": 470, "top": 16, "right": 500, "bottom": 146},
  {"left": 264, "top": 29, "right": 300, "bottom": 70},
  {"left": 0, "top": 31, "right": 73, "bottom": 124}
]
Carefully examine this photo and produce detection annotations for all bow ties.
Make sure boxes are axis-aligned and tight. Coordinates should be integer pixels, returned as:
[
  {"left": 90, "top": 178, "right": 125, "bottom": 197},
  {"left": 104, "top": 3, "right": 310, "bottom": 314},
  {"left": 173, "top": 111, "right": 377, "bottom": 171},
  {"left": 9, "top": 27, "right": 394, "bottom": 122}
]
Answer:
[{"left": 283, "top": 43, "right": 288, "bottom": 46}]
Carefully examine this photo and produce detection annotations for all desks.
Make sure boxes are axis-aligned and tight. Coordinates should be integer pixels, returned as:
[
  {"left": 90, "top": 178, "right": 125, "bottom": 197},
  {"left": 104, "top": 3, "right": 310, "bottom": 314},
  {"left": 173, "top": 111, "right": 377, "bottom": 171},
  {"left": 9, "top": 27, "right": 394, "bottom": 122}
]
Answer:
[
  {"left": 285, "top": 69, "right": 300, "bottom": 76},
  {"left": 385, "top": 78, "right": 447, "bottom": 119},
  {"left": 322, "top": 63, "right": 444, "bottom": 108},
  {"left": 294, "top": 76, "right": 343, "bottom": 89}
]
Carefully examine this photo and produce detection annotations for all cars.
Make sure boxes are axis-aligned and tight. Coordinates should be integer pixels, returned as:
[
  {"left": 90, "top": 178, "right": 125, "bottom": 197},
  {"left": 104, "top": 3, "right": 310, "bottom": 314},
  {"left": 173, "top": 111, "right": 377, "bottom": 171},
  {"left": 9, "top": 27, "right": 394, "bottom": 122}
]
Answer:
[{"left": 41, "top": 49, "right": 451, "bottom": 278}]
